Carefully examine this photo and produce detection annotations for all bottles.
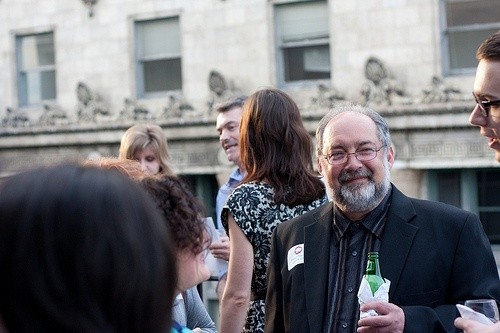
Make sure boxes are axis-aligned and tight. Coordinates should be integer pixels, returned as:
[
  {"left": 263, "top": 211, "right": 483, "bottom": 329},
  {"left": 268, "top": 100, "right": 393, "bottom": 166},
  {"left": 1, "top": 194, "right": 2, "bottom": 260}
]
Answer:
[{"left": 357, "top": 251, "right": 390, "bottom": 324}]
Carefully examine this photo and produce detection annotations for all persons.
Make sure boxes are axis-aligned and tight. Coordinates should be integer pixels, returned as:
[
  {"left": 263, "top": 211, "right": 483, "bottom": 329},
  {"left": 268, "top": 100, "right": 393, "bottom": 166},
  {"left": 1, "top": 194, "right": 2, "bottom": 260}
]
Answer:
[
  {"left": 264, "top": 104, "right": 500, "bottom": 333},
  {"left": 0, "top": 124, "right": 218, "bottom": 333},
  {"left": 453, "top": 31, "right": 500, "bottom": 333},
  {"left": 218, "top": 88, "right": 329, "bottom": 333},
  {"left": 209, "top": 95, "right": 249, "bottom": 302}
]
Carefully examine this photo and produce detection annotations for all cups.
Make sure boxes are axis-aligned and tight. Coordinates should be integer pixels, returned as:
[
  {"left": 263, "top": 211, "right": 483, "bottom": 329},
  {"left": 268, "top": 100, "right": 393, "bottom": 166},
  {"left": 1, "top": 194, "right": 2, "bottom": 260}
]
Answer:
[{"left": 465, "top": 300, "right": 499, "bottom": 324}]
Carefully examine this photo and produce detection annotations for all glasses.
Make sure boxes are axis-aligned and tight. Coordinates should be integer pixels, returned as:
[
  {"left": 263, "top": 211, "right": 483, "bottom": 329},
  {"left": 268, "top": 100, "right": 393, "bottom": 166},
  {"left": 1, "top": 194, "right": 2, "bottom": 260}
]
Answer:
[
  {"left": 319, "top": 145, "right": 387, "bottom": 165},
  {"left": 472, "top": 91, "right": 500, "bottom": 117}
]
